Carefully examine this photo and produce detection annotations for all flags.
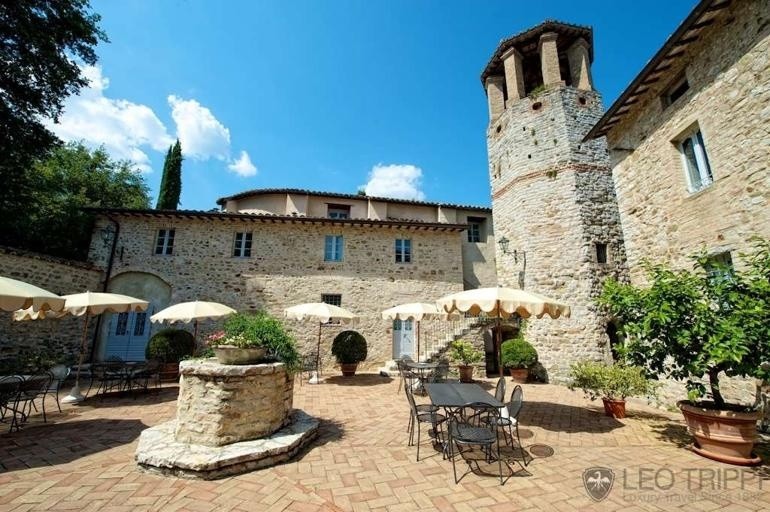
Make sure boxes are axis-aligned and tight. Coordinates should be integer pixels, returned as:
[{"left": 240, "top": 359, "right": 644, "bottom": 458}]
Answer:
[{"left": 209, "top": 345, "right": 239, "bottom": 362}]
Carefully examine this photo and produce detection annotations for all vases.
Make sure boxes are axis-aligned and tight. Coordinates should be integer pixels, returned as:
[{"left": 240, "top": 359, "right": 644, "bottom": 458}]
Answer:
[{"left": 204, "top": 329, "right": 251, "bottom": 349}]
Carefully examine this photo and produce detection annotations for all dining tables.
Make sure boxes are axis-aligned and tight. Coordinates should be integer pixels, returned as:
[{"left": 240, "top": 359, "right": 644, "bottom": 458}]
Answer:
[
  {"left": 198, "top": 308, "right": 308, "bottom": 376},
  {"left": 450, "top": 340, "right": 485, "bottom": 384},
  {"left": 330, "top": 330, "right": 368, "bottom": 377},
  {"left": 591, "top": 236, "right": 770, "bottom": 465},
  {"left": 145, "top": 328, "right": 193, "bottom": 382},
  {"left": 105, "top": 356, "right": 125, "bottom": 371},
  {"left": 498, "top": 339, "right": 537, "bottom": 385},
  {"left": 567, "top": 350, "right": 661, "bottom": 419}
]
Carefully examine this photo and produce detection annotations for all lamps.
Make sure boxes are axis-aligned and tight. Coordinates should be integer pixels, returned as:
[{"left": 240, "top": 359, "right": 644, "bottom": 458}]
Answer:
[
  {"left": 395, "top": 355, "right": 534, "bottom": 486},
  {"left": 0, "top": 354, "right": 165, "bottom": 437}
]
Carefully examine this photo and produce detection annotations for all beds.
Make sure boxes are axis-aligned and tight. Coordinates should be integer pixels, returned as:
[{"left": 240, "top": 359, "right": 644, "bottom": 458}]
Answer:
[
  {"left": 150, "top": 299, "right": 238, "bottom": 358},
  {"left": 435, "top": 285, "right": 571, "bottom": 405},
  {"left": 12, "top": 289, "right": 150, "bottom": 387},
  {"left": 380, "top": 302, "right": 461, "bottom": 373},
  {"left": 284, "top": 302, "right": 360, "bottom": 371},
  {"left": 0, "top": 276, "right": 66, "bottom": 312}
]
